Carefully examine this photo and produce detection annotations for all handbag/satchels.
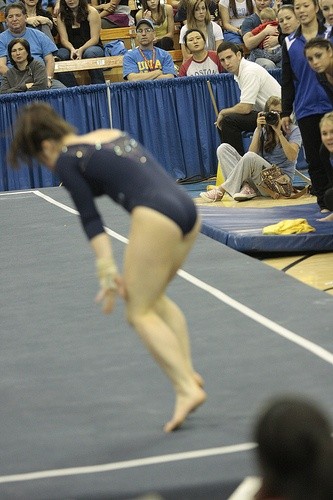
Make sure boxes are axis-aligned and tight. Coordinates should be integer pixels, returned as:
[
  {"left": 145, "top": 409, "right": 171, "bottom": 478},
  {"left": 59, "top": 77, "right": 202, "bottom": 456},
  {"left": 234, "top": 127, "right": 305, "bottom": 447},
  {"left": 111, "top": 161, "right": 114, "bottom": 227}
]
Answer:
[
  {"left": 103, "top": 39, "right": 127, "bottom": 57},
  {"left": 260, "top": 166, "right": 292, "bottom": 197}
]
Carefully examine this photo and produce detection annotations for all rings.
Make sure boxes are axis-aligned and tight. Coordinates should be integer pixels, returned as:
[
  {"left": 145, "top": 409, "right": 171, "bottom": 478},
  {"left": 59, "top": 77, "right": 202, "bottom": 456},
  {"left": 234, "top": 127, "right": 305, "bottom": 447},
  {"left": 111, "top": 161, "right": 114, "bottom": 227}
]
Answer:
[{"left": 111, "top": 9, "right": 113, "bottom": 11}]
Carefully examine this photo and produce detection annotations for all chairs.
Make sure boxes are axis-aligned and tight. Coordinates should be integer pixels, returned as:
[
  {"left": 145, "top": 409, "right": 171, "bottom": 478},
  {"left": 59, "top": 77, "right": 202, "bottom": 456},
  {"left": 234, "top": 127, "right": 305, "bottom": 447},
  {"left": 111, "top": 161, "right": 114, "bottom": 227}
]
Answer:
[{"left": 242, "top": 110, "right": 311, "bottom": 184}]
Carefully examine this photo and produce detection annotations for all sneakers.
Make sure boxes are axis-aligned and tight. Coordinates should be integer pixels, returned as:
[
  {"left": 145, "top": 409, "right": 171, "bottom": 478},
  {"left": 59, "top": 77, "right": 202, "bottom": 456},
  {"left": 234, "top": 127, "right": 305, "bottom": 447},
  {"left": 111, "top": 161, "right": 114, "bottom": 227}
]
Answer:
[
  {"left": 233, "top": 186, "right": 257, "bottom": 201},
  {"left": 199, "top": 189, "right": 224, "bottom": 202}
]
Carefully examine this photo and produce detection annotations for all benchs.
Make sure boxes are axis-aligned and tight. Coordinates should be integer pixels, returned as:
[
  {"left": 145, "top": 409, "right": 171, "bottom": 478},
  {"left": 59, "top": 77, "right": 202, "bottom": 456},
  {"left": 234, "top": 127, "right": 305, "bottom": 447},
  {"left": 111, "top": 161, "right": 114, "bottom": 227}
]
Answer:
[{"left": 55, "top": 18, "right": 254, "bottom": 84}]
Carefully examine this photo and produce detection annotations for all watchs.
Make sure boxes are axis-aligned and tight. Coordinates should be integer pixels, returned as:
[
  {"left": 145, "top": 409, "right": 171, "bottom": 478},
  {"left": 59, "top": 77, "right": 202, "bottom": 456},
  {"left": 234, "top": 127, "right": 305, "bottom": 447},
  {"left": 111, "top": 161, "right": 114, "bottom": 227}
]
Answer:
[{"left": 48, "top": 77, "right": 53, "bottom": 82}]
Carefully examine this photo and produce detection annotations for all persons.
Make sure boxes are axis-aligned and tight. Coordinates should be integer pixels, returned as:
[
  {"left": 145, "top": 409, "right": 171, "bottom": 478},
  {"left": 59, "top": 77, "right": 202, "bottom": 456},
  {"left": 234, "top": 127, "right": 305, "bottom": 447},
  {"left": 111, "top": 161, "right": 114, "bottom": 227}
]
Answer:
[
  {"left": 252, "top": 400, "right": 333, "bottom": 500},
  {"left": 278, "top": 4, "right": 300, "bottom": 43},
  {"left": 0, "top": 0, "right": 129, "bottom": 87},
  {"left": 318, "top": 0, "right": 333, "bottom": 28},
  {"left": 11, "top": 103, "right": 207, "bottom": 434},
  {"left": 303, "top": 37, "right": 333, "bottom": 104},
  {"left": 0, "top": 3, "right": 67, "bottom": 89},
  {"left": 281, "top": 0, "right": 333, "bottom": 214},
  {"left": 249, "top": 7, "right": 282, "bottom": 63},
  {"left": 178, "top": 0, "right": 224, "bottom": 56},
  {"left": 213, "top": 42, "right": 297, "bottom": 156},
  {"left": 200, "top": 96, "right": 302, "bottom": 202},
  {"left": 242, "top": 0, "right": 282, "bottom": 69},
  {"left": 180, "top": 29, "right": 226, "bottom": 77},
  {"left": 316, "top": 112, "right": 333, "bottom": 222},
  {"left": 218, "top": 0, "right": 257, "bottom": 46},
  {"left": 0, "top": 38, "right": 48, "bottom": 93},
  {"left": 128, "top": 0, "right": 221, "bottom": 51},
  {"left": 122, "top": 19, "right": 178, "bottom": 80}
]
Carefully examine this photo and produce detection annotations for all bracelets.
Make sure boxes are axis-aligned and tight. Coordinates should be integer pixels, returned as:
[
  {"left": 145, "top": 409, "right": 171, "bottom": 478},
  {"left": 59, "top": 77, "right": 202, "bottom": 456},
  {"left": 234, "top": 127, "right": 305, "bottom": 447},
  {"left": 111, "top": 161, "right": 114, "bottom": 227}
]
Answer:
[{"left": 237, "top": 29, "right": 240, "bottom": 34}]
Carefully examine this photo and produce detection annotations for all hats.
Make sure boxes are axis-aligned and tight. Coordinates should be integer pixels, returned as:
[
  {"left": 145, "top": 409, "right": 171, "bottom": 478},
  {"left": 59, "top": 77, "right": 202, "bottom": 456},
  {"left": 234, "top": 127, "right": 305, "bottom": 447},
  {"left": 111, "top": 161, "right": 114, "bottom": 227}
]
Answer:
[{"left": 136, "top": 19, "right": 155, "bottom": 31}]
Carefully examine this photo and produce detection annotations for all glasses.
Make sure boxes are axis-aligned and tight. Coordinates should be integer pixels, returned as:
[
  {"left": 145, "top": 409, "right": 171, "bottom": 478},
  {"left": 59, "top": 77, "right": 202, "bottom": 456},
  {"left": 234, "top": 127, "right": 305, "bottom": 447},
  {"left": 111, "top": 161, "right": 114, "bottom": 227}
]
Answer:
[{"left": 136, "top": 29, "right": 154, "bottom": 34}]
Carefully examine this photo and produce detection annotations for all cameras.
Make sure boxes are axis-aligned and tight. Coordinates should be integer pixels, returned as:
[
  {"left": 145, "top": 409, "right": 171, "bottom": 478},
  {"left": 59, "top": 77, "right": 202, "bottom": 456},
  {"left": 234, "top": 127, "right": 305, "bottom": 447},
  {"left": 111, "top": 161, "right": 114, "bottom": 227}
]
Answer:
[{"left": 260, "top": 111, "right": 280, "bottom": 127}]
